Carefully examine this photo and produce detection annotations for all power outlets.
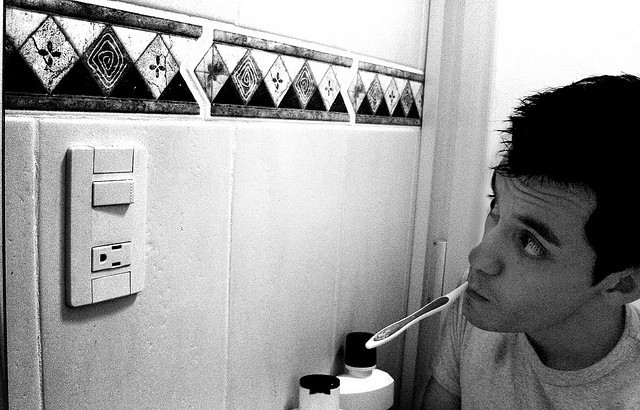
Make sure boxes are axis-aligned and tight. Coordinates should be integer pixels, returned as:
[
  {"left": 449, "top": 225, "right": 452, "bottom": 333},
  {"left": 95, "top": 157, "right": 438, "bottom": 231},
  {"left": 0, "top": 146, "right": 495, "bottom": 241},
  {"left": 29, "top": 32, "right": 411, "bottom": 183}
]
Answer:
[{"left": 65, "top": 147, "right": 143, "bottom": 307}]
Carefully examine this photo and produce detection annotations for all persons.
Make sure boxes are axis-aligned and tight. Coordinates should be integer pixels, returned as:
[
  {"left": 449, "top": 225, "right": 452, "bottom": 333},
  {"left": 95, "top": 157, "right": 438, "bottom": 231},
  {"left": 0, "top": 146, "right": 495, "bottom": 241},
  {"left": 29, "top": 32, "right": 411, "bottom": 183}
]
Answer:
[{"left": 424, "top": 74, "right": 640, "bottom": 410}]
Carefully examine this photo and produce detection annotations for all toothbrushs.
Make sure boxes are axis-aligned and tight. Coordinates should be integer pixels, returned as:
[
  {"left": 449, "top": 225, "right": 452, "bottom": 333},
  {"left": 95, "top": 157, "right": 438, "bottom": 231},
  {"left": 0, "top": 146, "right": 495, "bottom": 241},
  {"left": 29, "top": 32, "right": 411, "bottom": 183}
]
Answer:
[{"left": 364, "top": 280, "right": 469, "bottom": 355}]
